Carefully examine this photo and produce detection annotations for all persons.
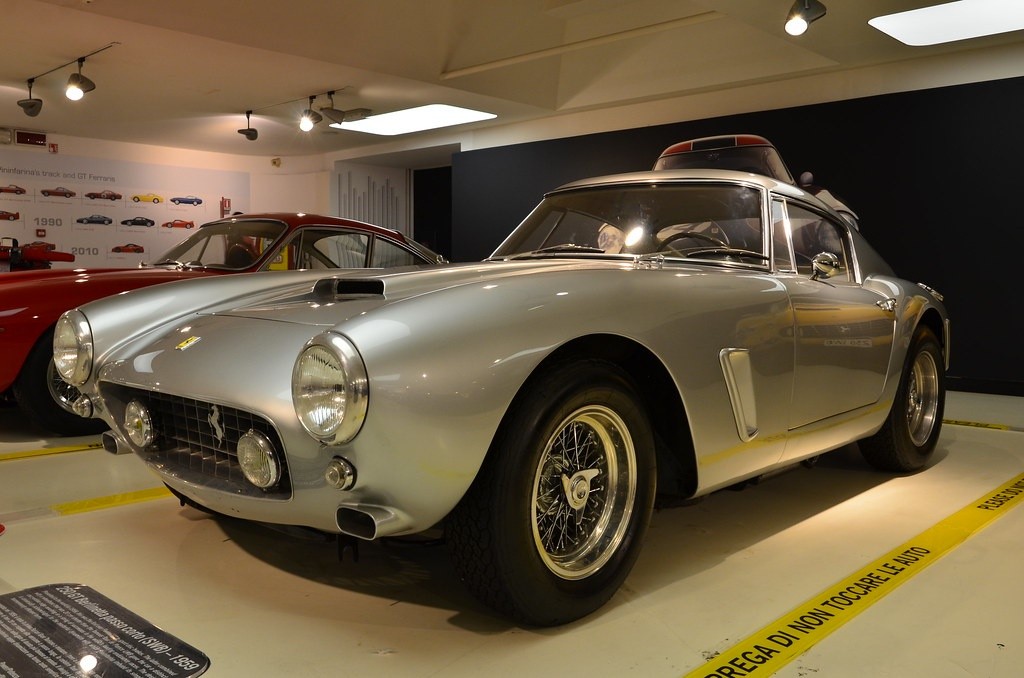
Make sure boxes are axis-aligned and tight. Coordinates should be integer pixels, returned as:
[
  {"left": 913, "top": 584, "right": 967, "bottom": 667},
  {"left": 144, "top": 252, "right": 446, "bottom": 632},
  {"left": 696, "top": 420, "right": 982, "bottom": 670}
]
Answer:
[{"left": 225, "top": 212, "right": 259, "bottom": 256}]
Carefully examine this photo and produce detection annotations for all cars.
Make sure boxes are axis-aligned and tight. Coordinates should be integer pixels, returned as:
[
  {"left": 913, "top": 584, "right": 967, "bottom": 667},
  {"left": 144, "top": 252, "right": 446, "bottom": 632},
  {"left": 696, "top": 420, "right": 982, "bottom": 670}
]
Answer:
[
  {"left": 76, "top": 215, "right": 113, "bottom": 225},
  {"left": 0, "top": 213, "right": 449, "bottom": 438},
  {"left": 0, "top": 185, "right": 26, "bottom": 195},
  {"left": 59, "top": 134, "right": 950, "bottom": 628},
  {"left": 169, "top": 195, "right": 202, "bottom": 206},
  {"left": 112, "top": 243, "right": 144, "bottom": 254},
  {"left": 84, "top": 189, "right": 123, "bottom": 201},
  {"left": 121, "top": 216, "right": 155, "bottom": 227},
  {"left": 41, "top": 187, "right": 76, "bottom": 198},
  {"left": 0, "top": 211, "right": 19, "bottom": 221},
  {"left": 131, "top": 192, "right": 164, "bottom": 204},
  {"left": 162, "top": 219, "right": 194, "bottom": 229}
]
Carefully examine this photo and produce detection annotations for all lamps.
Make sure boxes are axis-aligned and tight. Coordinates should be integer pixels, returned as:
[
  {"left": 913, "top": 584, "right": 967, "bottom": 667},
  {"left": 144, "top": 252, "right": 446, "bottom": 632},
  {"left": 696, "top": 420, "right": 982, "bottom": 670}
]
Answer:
[
  {"left": 16, "top": 83, "right": 43, "bottom": 117},
  {"left": 238, "top": 112, "right": 258, "bottom": 141},
  {"left": 782, "top": 0, "right": 828, "bottom": 37},
  {"left": 320, "top": 92, "right": 345, "bottom": 125},
  {"left": 299, "top": 98, "right": 321, "bottom": 131},
  {"left": 67, "top": 59, "right": 97, "bottom": 100}
]
